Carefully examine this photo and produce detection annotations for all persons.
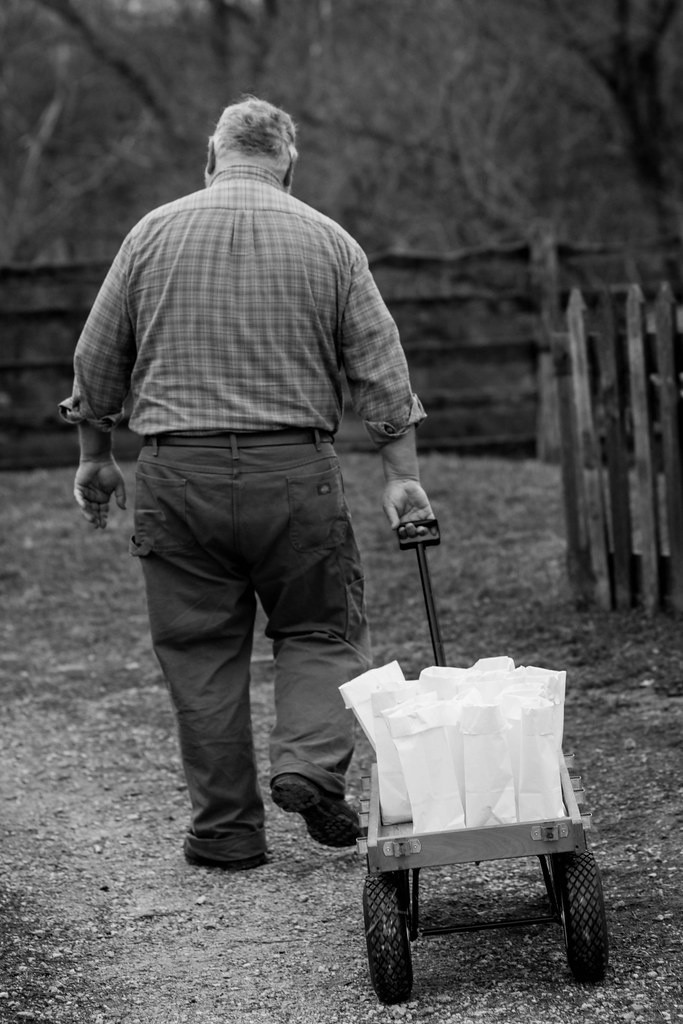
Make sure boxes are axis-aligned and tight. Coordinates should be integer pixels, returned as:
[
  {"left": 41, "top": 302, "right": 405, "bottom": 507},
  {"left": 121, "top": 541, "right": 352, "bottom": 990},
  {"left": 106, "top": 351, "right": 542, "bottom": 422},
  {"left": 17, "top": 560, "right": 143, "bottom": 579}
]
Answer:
[{"left": 61, "top": 99, "right": 439, "bottom": 871}]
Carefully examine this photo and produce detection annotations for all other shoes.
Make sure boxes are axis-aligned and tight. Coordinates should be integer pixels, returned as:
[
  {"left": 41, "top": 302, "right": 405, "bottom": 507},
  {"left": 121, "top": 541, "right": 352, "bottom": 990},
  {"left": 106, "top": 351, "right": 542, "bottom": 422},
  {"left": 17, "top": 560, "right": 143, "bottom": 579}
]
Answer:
[
  {"left": 182, "top": 835, "right": 268, "bottom": 871},
  {"left": 272, "top": 774, "right": 362, "bottom": 847}
]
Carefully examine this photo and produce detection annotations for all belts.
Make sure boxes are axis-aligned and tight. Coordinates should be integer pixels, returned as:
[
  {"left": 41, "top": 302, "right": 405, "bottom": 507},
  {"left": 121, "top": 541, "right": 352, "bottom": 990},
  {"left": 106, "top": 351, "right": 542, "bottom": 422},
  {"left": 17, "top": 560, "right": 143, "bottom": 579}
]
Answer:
[{"left": 143, "top": 430, "right": 334, "bottom": 448}]
